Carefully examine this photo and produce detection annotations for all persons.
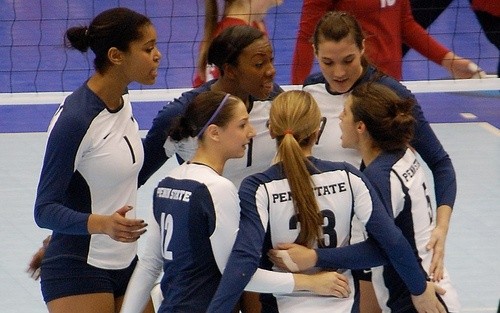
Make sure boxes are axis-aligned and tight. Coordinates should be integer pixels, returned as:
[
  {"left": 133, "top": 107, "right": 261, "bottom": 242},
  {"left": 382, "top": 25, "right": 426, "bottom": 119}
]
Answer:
[
  {"left": 300, "top": 12, "right": 458, "bottom": 313},
  {"left": 208, "top": 90, "right": 447, "bottom": 313},
  {"left": 269, "top": 84, "right": 447, "bottom": 313},
  {"left": 35, "top": 7, "right": 161, "bottom": 313},
  {"left": 193, "top": 0, "right": 495, "bottom": 86},
  {"left": 119, "top": 92, "right": 350, "bottom": 313},
  {"left": 25, "top": 26, "right": 284, "bottom": 313}
]
